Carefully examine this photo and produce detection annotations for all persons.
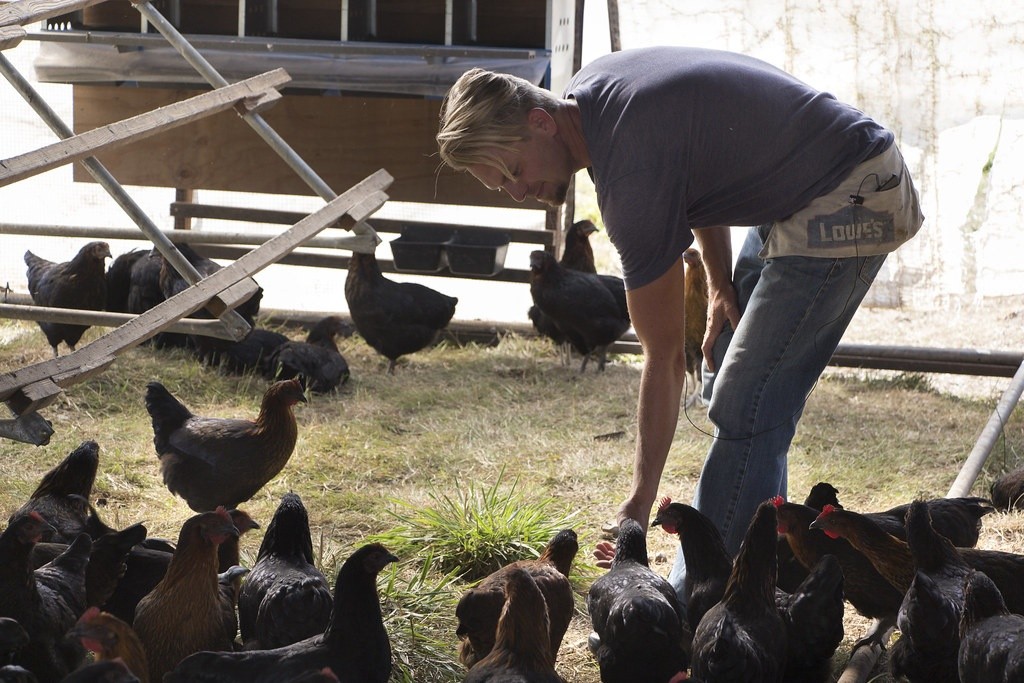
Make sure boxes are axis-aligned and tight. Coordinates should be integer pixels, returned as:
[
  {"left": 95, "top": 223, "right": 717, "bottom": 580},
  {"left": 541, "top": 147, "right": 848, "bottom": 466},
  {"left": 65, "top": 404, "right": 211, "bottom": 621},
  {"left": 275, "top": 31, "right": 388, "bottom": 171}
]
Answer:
[{"left": 437, "top": 45, "right": 925, "bottom": 614}]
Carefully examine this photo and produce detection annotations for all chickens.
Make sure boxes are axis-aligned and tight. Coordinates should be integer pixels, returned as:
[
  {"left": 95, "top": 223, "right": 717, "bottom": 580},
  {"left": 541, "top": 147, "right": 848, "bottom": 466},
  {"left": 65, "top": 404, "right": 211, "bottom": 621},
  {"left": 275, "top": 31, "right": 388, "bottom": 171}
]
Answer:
[{"left": 0, "top": 218, "right": 1023, "bottom": 682}]
